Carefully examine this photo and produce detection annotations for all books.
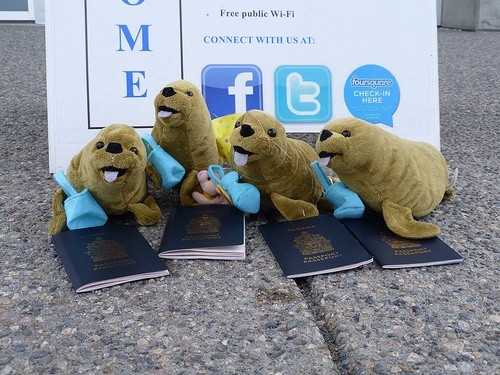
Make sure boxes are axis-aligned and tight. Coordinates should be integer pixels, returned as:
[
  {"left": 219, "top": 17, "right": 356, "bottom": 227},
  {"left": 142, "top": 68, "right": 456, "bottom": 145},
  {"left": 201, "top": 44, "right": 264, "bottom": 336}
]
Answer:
[
  {"left": 340, "top": 217, "right": 463, "bottom": 269},
  {"left": 158, "top": 206, "right": 246, "bottom": 260},
  {"left": 52, "top": 225, "right": 169, "bottom": 294},
  {"left": 258, "top": 216, "right": 373, "bottom": 278}
]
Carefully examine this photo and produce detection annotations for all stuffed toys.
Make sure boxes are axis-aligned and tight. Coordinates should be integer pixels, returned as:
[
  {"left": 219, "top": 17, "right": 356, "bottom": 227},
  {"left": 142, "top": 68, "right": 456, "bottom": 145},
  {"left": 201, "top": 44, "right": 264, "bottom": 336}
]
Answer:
[
  {"left": 47, "top": 123, "right": 161, "bottom": 236},
  {"left": 146, "top": 80, "right": 221, "bottom": 204},
  {"left": 316, "top": 118, "right": 448, "bottom": 238},
  {"left": 192, "top": 170, "right": 229, "bottom": 204},
  {"left": 228, "top": 111, "right": 322, "bottom": 221}
]
